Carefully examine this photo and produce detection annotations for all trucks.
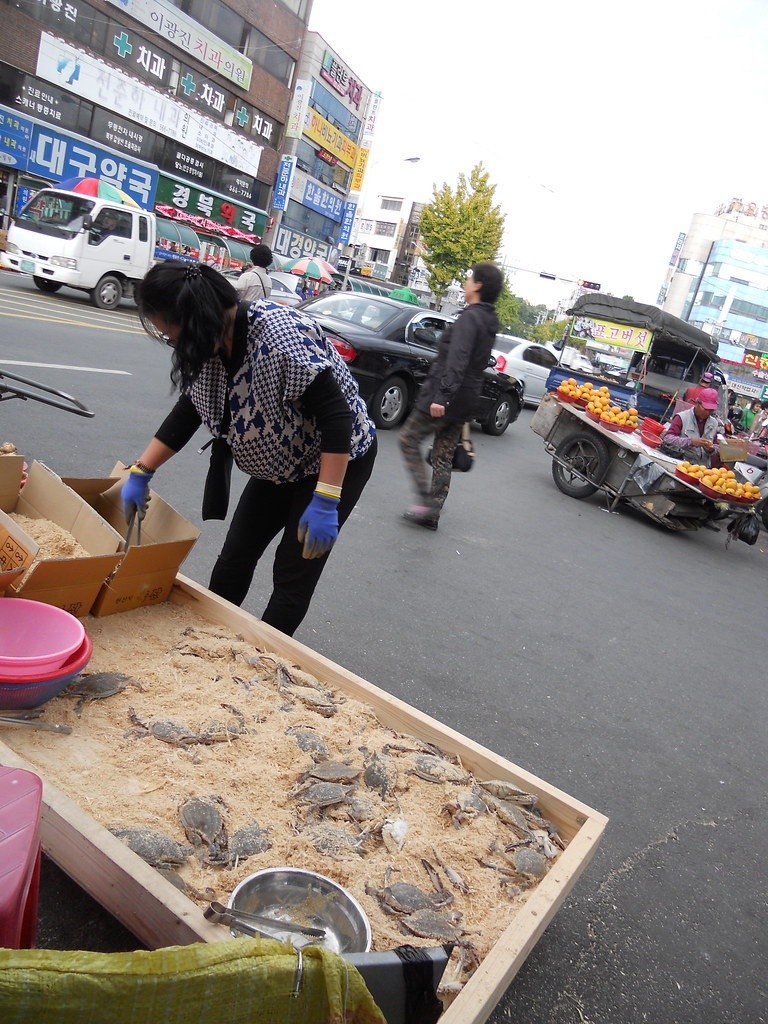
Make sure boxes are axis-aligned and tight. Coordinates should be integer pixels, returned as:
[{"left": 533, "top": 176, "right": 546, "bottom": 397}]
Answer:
[
  {"left": 545, "top": 294, "right": 737, "bottom": 426},
  {"left": 1, "top": 188, "right": 229, "bottom": 311}
]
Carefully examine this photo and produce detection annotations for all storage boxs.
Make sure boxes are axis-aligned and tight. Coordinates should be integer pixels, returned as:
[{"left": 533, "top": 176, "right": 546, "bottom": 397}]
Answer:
[
  {"left": 61, "top": 459, "right": 202, "bottom": 619},
  {"left": 0, "top": 456, "right": 127, "bottom": 618}
]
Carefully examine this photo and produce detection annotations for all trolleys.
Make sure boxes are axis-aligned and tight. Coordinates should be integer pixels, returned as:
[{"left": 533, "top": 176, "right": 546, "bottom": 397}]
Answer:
[{"left": 530, "top": 393, "right": 756, "bottom": 533}]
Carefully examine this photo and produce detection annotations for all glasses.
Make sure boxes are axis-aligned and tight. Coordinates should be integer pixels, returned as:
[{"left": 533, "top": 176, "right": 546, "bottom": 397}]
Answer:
[{"left": 159, "top": 333, "right": 176, "bottom": 350}]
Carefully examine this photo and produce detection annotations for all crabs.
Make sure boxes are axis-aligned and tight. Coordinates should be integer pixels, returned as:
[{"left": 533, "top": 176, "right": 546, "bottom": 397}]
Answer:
[{"left": 54, "top": 626, "right": 569, "bottom": 994}]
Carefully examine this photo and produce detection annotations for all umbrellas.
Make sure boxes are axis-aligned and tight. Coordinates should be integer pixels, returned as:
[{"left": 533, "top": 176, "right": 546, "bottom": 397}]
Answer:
[
  {"left": 300, "top": 257, "right": 339, "bottom": 275},
  {"left": 116, "top": 188, "right": 142, "bottom": 209},
  {"left": 53, "top": 177, "right": 123, "bottom": 204},
  {"left": 275, "top": 259, "right": 336, "bottom": 286}
]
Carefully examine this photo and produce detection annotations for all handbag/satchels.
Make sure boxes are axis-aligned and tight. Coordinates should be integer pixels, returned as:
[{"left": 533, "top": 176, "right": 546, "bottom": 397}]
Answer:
[
  {"left": 426, "top": 422, "right": 474, "bottom": 471},
  {"left": 198, "top": 438, "right": 234, "bottom": 521},
  {"left": 759, "top": 437, "right": 768, "bottom": 445},
  {"left": 745, "top": 427, "right": 749, "bottom": 433}
]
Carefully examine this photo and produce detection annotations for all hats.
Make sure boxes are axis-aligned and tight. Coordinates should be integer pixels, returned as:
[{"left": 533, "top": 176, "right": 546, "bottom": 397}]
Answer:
[
  {"left": 703, "top": 373, "right": 713, "bottom": 382},
  {"left": 698, "top": 388, "right": 718, "bottom": 410}
]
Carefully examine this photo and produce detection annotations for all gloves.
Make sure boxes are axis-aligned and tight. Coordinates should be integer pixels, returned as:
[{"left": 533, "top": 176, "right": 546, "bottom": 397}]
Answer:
[
  {"left": 298, "top": 495, "right": 338, "bottom": 560},
  {"left": 121, "top": 472, "right": 153, "bottom": 527}
]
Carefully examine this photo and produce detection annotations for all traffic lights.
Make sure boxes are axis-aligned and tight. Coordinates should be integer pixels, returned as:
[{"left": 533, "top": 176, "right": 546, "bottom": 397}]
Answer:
[{"left": 583, "top": 281, "right": 600, "bottom": 291}]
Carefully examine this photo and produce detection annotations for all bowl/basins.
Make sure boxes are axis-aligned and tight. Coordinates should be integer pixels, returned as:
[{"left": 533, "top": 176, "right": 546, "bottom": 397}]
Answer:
[
  {"left": 574, "top": 397, "right": 589, "bottom": 406},
  {"left": 675, "top": 468, "right": 699, "bottom": 485},
  {"left": 641, "top": 416, "right": 665, "bottom": 438},
  {"left": 632, "top": 372, "right": 644, "bottom": 380},
  {"left": 725, "top": 491, "right": 742, "bottom": 500},
  {"left": 641, "top": 430, "right": 662, "bottom": 448},
  {"left": 556, "top": 387, "right": 577, "bottom": 402},
  {"left": 585, "top": 406, "right": 601, "bottom": 422},
  {"left": 599, "top": 419, "right": 623, "bottom": 431},
  {"left": 739, "top": 494, "right": 754, "bottom": 503},
  {"left": 620, "top": 423, "right": 636, "bottom": 433},
  {"left": 751, "top": 496, "right": 762, "bottom": 503},
  {"left": 699, "top": 479, "right": 726, "bottom": 498}
]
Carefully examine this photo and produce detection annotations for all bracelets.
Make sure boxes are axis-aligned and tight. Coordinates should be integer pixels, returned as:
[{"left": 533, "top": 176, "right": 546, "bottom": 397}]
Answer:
[{"left": 135, "top": 460, "right": 156, "bottom": 474}]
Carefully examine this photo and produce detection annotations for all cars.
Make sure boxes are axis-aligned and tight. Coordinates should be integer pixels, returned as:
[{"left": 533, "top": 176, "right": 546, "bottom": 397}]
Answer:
[
  {"left": 490, "top": 332, "right": 559, "bottom": 407},
  {"left": 294, "top": 292, "right": 523, "bottom": 436},
  {"left": 218, "top": 269, "right": 303, "bottom": 308}
]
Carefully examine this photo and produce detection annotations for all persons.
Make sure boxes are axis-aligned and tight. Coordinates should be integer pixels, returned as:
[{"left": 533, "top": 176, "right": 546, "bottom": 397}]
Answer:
[
  {"left": 121, "top": 257, "right": 379, "bottom": 637},
  {"left": 102, "top": 212, "right": 125, "bottom": 237},
  {"left": 396, "top": 262, "right": 504, "bottom": 532},
  {"left": 684, "top": 372, "right": 714, "bottom": 405},
  {"left": 233, "top": 243, "right": 273, "bottom": 302},
  {"left": 749, "top": 401, "right": 768, "bottom": 438},
  {"left": 739, "top": 399, "right": 762, "bottom": 432},
  {"left": 663, "top": 388, "right": 721, "bottom": 532}
]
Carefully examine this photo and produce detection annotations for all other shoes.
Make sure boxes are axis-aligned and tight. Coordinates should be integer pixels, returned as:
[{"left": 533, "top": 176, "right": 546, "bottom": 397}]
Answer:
[{"left": 404, "top": 507, "right": 438, "bottom": 529}]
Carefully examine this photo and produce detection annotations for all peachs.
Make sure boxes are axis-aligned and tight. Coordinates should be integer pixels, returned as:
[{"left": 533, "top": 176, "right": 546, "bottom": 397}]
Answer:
[
  {"left": 677, "top": 461, "right": 762, "bottom": 501},
  {"left": 556, "top": 377, "right": 639, "bottom": 429}
]
